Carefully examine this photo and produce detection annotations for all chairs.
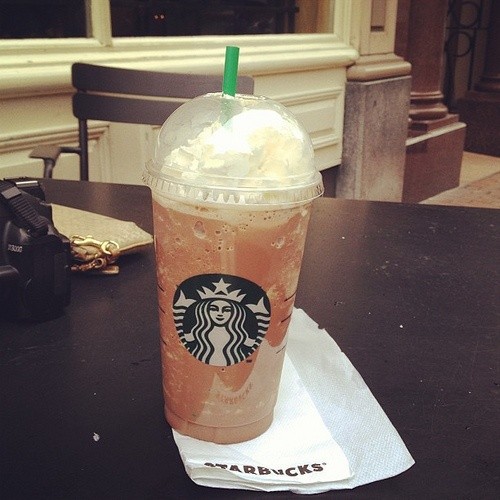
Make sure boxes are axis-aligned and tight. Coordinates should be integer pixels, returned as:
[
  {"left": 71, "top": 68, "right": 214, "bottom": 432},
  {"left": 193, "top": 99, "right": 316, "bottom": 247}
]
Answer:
[{"left": 27, "top": 62, "right": 255, "bottom": 183}]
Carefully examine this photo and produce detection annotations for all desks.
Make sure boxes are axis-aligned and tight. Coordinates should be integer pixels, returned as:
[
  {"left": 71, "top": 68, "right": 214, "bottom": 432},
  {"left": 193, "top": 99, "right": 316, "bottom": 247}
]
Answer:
[{"left": 0, "top": 175, "right": 499, "bottom": 500}]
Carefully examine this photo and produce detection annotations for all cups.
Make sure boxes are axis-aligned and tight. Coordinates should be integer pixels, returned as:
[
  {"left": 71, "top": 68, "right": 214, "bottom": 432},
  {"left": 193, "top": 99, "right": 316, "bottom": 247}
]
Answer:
[{"left": 139, "top": 91, "right": 326, "bottom": 444}]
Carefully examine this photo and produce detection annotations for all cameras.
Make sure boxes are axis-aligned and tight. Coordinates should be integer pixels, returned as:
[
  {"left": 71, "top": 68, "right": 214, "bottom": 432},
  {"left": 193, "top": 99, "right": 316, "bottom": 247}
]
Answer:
[{"left": 0, "top": 179, "right": 74, "bottom": 322}]
